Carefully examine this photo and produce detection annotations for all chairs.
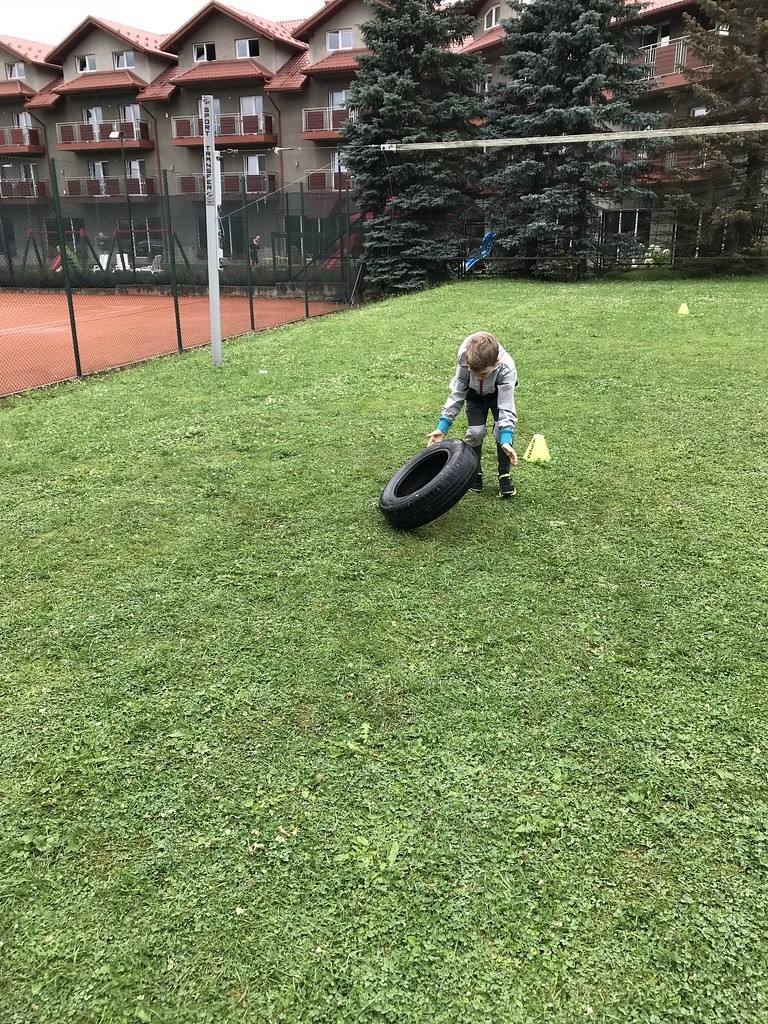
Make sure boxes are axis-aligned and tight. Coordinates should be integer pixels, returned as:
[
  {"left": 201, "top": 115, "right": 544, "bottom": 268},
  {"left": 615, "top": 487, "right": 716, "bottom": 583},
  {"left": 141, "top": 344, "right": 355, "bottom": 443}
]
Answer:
[
  {"left": 113, "top": 253, "right": 132, "bottom": 272},
  {"left": 135, "top": 254, "right": 163, "bottom": 275},
  {"left": 93, "top": 254, "right": 109, "bottom": 271}
]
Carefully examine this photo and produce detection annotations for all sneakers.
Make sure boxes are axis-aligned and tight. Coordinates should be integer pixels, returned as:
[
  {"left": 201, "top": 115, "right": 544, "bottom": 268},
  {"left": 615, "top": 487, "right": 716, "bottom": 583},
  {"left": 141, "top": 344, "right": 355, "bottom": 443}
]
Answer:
[
  {"left": 468, "top": 470, "right": 483, "bottom": 494},
  {"left": 497, "top": 473, "right": 516, "bottom": 498}
]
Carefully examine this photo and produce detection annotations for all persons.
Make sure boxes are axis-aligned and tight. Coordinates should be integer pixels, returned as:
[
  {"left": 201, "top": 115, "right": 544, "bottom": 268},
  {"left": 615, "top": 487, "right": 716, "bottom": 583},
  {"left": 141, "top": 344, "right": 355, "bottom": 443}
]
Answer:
[
  {"left": 427, "top": 330, "right": 518, "bottom": 496},
  {"left": 251, "top": 235, "right": 260, "bottom": 266}
]
